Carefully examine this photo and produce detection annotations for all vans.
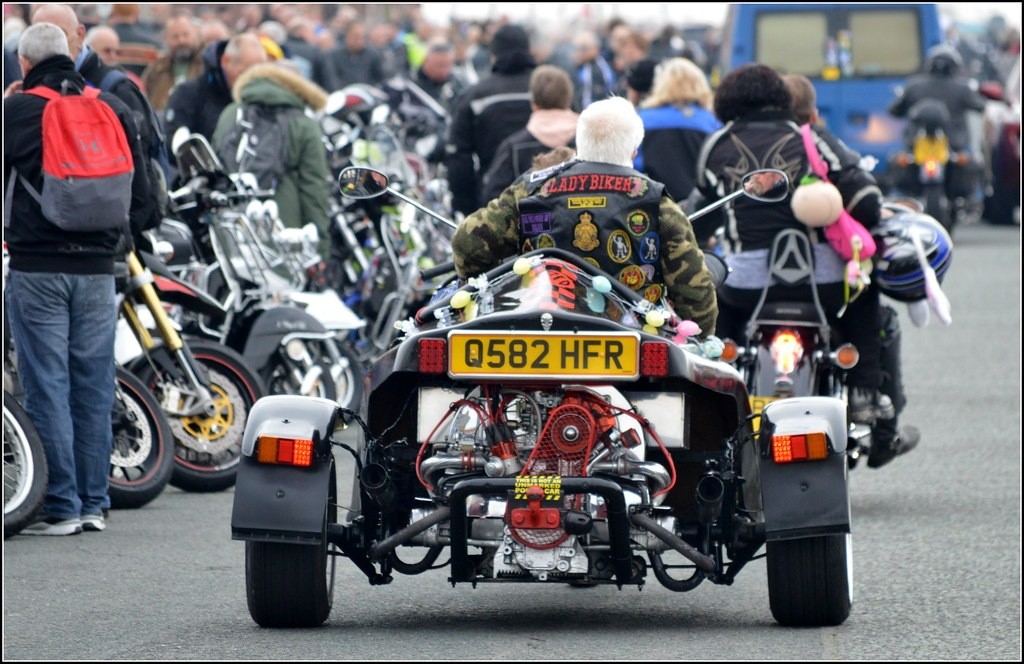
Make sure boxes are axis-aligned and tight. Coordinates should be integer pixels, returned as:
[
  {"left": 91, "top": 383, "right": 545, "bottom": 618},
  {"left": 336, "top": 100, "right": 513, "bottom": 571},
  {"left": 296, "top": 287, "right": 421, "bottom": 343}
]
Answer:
[{"left": 718, "top": 1, "right": 945, "bottom": 184}]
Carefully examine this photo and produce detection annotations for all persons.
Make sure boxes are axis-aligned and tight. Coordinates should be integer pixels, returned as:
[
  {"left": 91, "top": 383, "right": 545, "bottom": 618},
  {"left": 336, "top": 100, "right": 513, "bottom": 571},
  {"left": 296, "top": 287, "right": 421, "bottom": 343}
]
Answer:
[
  {"left": 452, "top": 97, "right": 720, "bottom": 339},
  {"left": 0, "top": 1, "right": 1020, "bottom": 541}
]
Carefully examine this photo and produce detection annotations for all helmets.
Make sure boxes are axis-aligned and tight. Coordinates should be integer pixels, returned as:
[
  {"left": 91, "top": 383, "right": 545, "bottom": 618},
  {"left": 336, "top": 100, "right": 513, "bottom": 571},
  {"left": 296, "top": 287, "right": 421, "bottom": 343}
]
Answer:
[
  {"left": 872, "top": 203, "right": 954, "bottom": 303},
  {"left": 929, "top": 55, "right": 956, "bottom": 79}
]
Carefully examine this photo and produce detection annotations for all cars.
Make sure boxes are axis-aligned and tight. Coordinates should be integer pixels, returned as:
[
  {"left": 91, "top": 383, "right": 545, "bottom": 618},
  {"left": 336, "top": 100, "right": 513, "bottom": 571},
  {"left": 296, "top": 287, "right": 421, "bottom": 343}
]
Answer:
[{"left": 979, "top": 59, "right": 1022, "bottom": 227}]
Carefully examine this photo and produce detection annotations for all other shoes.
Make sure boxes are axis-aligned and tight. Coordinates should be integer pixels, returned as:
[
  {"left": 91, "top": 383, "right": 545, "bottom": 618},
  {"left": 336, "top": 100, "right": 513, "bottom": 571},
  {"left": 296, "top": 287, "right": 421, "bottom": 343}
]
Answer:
[{"left": 868, "top": 419, "right": 921, "bottom": 469}]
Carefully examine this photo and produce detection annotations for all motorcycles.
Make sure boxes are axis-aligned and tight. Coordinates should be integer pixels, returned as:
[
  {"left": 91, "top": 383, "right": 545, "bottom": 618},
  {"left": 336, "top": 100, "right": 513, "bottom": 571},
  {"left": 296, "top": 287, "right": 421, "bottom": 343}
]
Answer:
[
  {"left": 2, "top": 75, "right": 897, "bottom": 542},
  {"left": 231, "top": 166, "right": 856, "bottom": 629},
  {"left": 894, "top": 75, "right": 979, "bottom": 234}
]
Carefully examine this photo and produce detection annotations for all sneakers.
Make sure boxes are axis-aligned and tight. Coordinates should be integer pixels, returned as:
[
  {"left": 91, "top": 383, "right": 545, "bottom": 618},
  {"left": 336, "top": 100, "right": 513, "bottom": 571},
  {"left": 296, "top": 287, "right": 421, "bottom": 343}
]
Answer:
[
  {"left": 80, "top": 506, "right": 109, "bottom": 532},
  {"left": 17, "top": 512, "right": 83, "bottom": 536}
]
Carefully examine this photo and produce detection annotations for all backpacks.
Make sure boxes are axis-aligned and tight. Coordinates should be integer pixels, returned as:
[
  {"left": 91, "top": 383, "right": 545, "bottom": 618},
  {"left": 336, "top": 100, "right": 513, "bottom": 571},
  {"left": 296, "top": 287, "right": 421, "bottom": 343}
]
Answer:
[
  {"left": 215, "top": 103, "right": 295, "bottom": 190},
  {"left": 22, "top": 85, "right": 134, "bottom": 232},
  {"left": 101, "top": 67, "right": 167, "bottom": 233}
]
